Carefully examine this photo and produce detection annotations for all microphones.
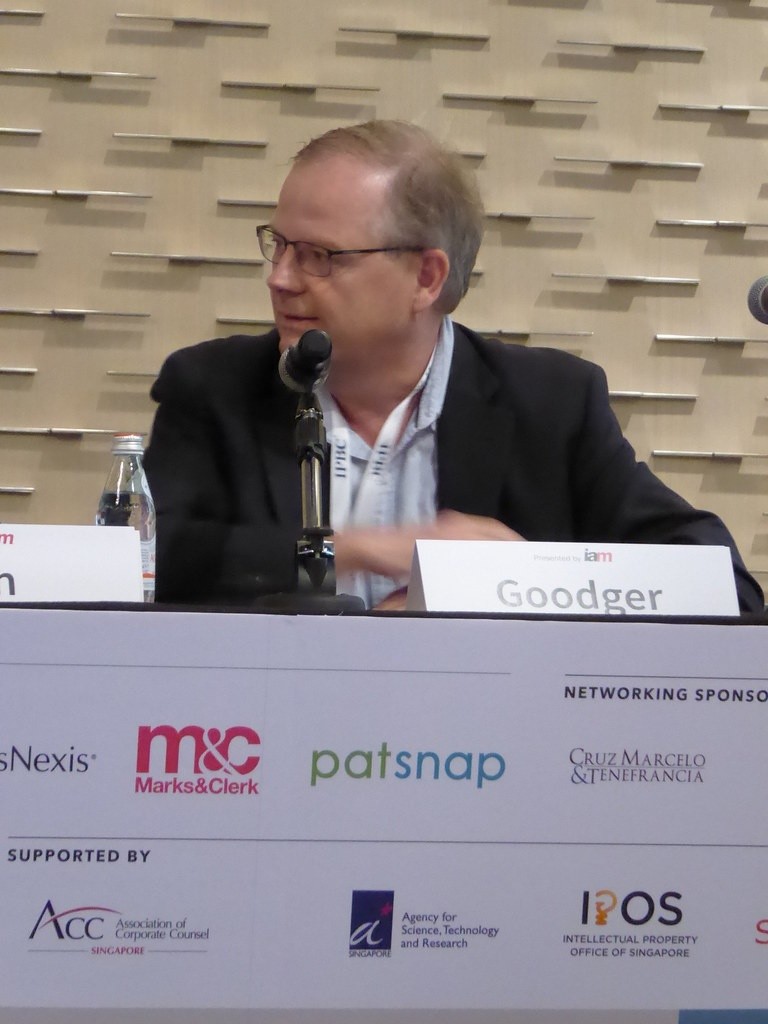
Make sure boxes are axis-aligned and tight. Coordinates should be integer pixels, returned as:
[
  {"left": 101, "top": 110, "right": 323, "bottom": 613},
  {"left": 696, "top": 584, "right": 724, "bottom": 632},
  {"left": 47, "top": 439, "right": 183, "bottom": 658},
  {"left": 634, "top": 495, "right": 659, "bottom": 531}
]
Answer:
[
  {"left": 748, "top": 276, "right": 768, "bottom": 326},
  {"left": 279, "top": 328, "right": 332, "bottom": 393}
]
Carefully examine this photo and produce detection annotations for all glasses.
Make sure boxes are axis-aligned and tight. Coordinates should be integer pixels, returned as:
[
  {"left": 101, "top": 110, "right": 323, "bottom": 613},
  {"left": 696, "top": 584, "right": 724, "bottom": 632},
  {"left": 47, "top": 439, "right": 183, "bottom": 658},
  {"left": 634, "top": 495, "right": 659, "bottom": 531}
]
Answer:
[{"left": 254, "top": 224, "right": 435, "bottom": 279}]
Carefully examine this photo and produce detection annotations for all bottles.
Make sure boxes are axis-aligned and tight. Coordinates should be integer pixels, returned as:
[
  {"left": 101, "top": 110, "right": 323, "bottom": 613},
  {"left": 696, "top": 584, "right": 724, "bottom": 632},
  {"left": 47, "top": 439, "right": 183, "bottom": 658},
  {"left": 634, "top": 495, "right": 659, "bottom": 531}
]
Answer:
[{"left": 96, "top": 435, "right": 156, "bottom": 602}]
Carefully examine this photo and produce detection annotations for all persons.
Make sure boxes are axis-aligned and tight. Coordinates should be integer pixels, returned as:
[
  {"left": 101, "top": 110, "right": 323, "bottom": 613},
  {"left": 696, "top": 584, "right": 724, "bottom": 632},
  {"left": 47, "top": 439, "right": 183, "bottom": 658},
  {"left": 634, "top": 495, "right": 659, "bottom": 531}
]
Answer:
[{"left": 142, "top": 119, "right": 766, "bottom": 625}]
